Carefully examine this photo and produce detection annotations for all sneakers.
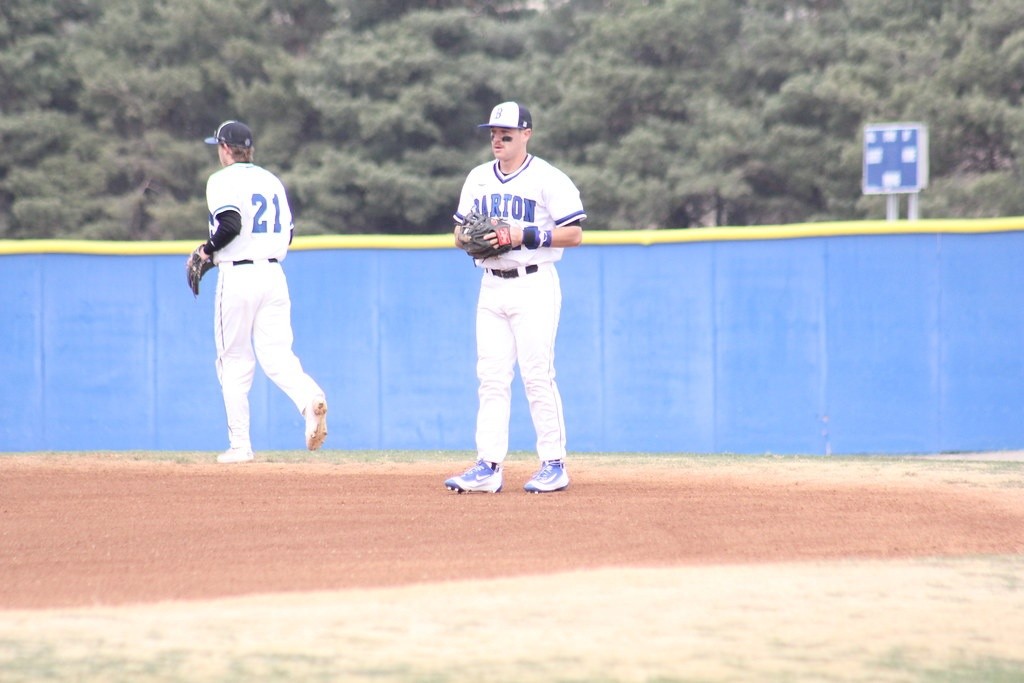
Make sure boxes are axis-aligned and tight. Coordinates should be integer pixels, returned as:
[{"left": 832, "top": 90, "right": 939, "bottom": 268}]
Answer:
[
  {"left": 445, "top": 458, "right": 503, "bottom": 494},
  {"left": 524, "top": 460, "right": 569, "bottom": 494},
  {"left": 216, "top": 447, "right": 253, "bottom": 463},
  {"left": 304, "top": 396, "right": 328, "bottom": 451}
]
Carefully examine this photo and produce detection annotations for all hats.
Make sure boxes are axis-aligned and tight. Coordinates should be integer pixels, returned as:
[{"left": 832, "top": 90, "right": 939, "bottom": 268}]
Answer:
[
  {"left": 204, "top": 120, "right": 253, "bottom": 148},
  {"left": 477, "top": 101, "right": 533, "bottom": 130}
]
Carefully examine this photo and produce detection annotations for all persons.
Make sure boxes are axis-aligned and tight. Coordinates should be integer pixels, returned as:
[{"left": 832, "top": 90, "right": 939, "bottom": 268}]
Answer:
[
  {"left": 186, "top": 120, "right": 328, "bottom": 463},
  {"left": 445, "top": 102, "right": 586, "bottom": 493}
]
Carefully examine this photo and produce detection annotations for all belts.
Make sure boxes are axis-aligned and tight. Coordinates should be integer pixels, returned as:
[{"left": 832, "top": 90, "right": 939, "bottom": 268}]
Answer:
[
  {"left": 486, "top": 264, "right": 538, "bottom": 279},
  {"left": 233, "top": 258, "right": 278, "bottom": 266}
]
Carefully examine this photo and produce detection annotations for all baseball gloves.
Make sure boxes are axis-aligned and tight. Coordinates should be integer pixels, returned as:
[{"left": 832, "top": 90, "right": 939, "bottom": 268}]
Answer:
[
  {"left": 186, "top": 244, "right": 215, "bottom": 295},
  {"left": 463, "top": 212, "right": 512, "bottom": 259}
]
